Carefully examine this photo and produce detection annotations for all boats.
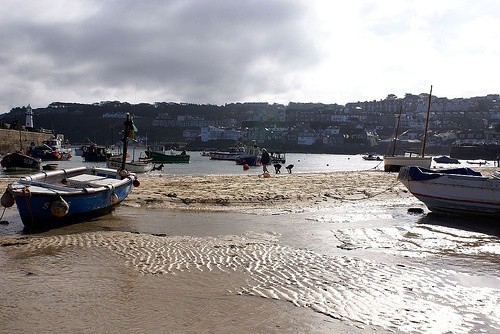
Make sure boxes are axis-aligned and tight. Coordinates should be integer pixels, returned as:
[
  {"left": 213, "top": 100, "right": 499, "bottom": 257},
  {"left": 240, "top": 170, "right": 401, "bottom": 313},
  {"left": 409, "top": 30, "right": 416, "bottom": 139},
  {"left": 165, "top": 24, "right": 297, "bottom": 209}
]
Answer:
[
  {"left": 82, "top": 137, "right": 124, "bottom": 162},
  {"left": 42, "top": 163, "right": 59, "bottom": 170},
  {"left": 382, "top": 84, "right": 434, "bottom": 172},
  {"left": 433, "top": 155, "right": 461, "bottom": 164},
  {"left": 5, "top": 111, "right": 137, "bottom": 232},
  {"left": 396, "top": 165, "right": 500, "bottom": 226},
  {"left": 144, "top": 144, "right": 191, "bottom": 164},
  {"left": 209, "top": 139, "right": 286, "bottom": 167},
  {"left": 0, "top": 128, "right": 41, "bottom": 173},
  {"left": 362, "top": 154, "right": 380, "bottom": 160},
  {"left": 27, "top": 136, "right": 73, "bottom": 161},
  {"left": 106, "top": 150, "right": 156, "bottom": 173}
]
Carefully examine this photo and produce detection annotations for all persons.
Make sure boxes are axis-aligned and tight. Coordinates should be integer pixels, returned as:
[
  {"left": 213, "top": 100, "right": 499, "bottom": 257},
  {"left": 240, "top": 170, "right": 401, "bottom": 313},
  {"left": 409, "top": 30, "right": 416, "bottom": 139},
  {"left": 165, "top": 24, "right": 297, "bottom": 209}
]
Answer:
[
  {"left": 258, "top": 148, "right": 271, "bottom": 174},
  {"left": 274, "top": 163, "right": 282, "bottom": 174},
  {"left": 285, "top": 164, "right": 294, "bottom": 173}
]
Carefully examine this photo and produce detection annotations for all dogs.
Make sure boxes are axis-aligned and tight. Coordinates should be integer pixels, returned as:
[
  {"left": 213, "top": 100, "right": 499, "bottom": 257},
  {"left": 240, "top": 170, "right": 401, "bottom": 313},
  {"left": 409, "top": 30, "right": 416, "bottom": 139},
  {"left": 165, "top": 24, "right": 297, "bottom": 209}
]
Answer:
[{"left": 151, "top": 163, "right": 164, "bottom": 171}]
